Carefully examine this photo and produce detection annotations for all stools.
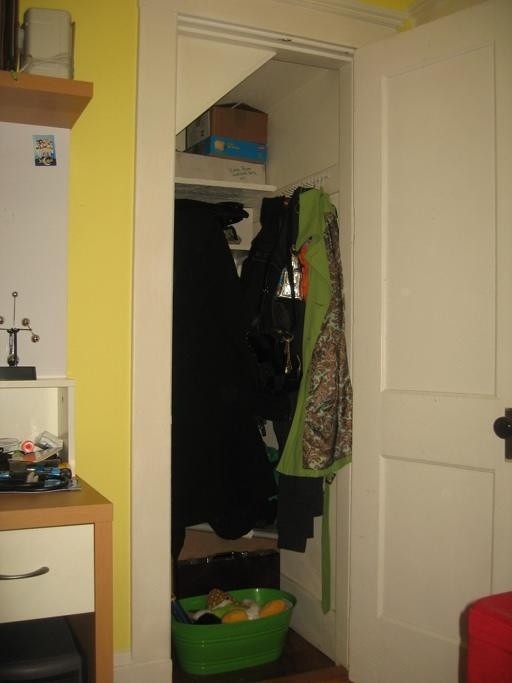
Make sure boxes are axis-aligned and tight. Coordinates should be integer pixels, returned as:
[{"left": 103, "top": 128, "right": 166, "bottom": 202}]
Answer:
[{"left": 1, "top": 616, "right": 88, "bottom": 682}]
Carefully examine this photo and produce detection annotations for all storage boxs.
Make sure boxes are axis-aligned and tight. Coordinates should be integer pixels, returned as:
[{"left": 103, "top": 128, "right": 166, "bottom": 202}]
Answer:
[
  {"left": 175, "top": 146, "right": 267, "bottom": 183},
  {"left": 184, "top": 101, "right": 268, "bottom": 146},
  {"left": 182, "top": 135, "right": 268, "bottom": 162}
]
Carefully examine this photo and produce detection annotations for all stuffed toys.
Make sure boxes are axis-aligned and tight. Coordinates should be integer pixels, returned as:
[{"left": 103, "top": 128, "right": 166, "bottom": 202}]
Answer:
[{"left": 191, "top": 586, "right": 287, "bottom": 625}]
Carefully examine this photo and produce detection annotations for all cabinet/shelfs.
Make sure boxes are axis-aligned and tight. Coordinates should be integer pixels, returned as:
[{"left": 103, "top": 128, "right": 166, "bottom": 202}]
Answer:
[{"left": 175, "top": 174, "right": 278, "bottom": 254}]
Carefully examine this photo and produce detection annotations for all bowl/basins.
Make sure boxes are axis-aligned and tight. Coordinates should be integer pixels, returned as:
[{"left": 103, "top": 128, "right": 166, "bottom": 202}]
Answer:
[{"left": 171, "top": 585, "right": 296, "bottom": 674}]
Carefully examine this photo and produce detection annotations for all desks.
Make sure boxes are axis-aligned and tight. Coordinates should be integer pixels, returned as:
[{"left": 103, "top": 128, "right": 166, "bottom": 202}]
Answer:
[{"left": 1, "top": 473, "right": 115, "bottom": 681}]
